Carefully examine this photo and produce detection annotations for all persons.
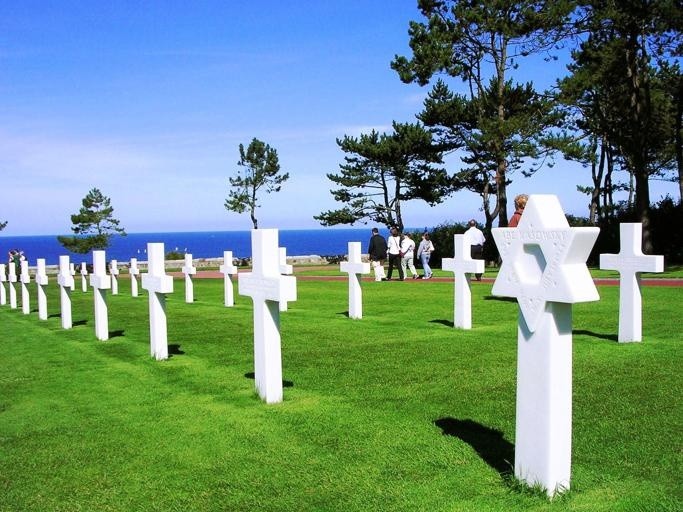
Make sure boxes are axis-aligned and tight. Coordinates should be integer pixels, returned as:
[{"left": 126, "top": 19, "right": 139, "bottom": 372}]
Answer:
[
  {"left": 400, "top": 231, "right": 419, "bottom": 281},
  {"left": 507, "top": 193, "right": 528, "bottom": 228},
  {"left": 465, "top": 220, "right": 486, "bottom": 281},
  {"left": 385, "top": 228, "right": 404, "bottom": 281},
  {"left": 8, "top": 250, "right": 13, "bottom": 261},
  {"left": 367, "top": 228, "right": 387, "bottom": 281},
  {"left": 14, "top": 249, "right": 20, "bottom": 268},
  {"left": 417, "top": 231, "right": 435, "bottom": 280},
  {"left": 19, "top": 251, "right": 25, "bottom": 261}
]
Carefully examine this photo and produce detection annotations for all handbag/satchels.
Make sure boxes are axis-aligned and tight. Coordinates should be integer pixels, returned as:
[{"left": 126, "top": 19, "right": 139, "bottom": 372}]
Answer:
[{"left": 398, "top": 249, "right": 404, "bottom": 258}]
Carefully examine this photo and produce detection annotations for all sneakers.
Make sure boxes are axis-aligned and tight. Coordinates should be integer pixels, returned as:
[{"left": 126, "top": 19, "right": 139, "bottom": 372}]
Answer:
[{"left": 374, "top": 272, "right": 433, "bottom": 281}]
